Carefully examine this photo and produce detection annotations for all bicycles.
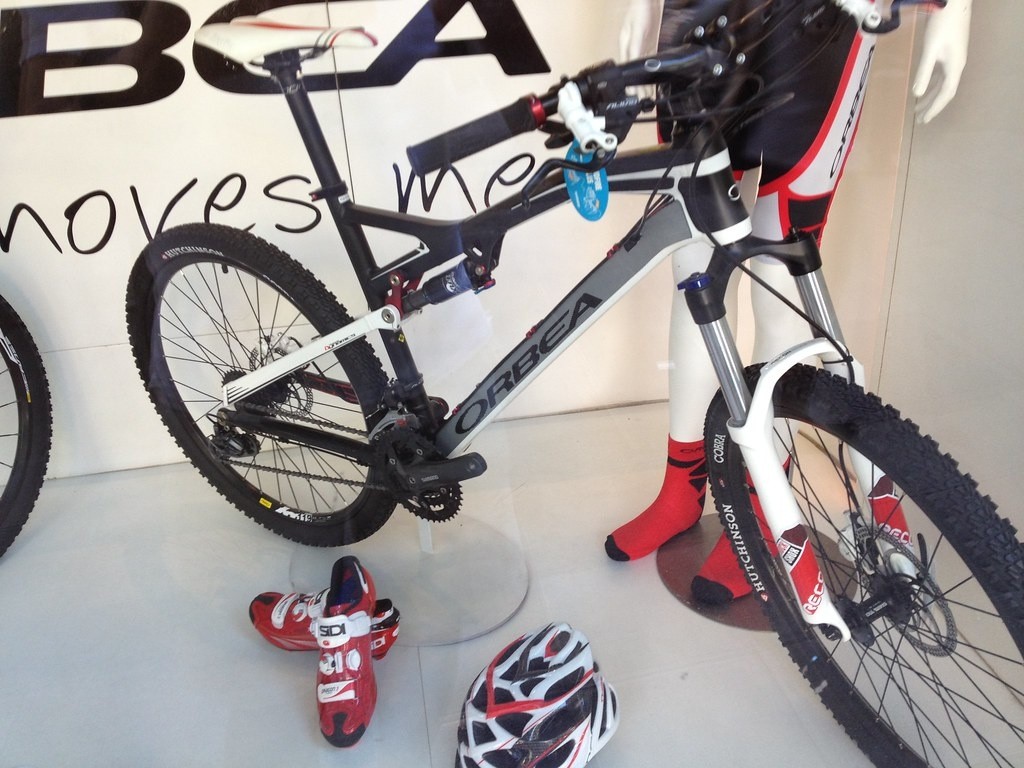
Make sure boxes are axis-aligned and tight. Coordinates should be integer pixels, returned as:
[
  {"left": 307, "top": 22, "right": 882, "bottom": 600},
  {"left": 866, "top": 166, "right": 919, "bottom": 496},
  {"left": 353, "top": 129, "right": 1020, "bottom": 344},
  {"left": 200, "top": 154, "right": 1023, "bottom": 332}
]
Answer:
[{"left": 120, "top": 2, "right": 1024, "bottom": 768}]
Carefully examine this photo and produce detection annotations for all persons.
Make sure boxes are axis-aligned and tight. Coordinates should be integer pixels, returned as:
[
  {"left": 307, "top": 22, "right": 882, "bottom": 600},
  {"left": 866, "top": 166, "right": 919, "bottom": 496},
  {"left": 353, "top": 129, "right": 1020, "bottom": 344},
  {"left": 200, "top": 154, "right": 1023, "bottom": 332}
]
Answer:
[{"left": 604, "top": 1, "right": 969, "bottom": 606}]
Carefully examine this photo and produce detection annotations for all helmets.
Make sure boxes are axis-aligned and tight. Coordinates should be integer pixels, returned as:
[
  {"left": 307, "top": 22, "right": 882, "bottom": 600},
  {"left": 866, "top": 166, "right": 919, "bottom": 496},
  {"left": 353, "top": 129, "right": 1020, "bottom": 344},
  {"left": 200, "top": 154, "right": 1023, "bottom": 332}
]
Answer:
[{"left": 453, "top": 620, "right": 618, "bottom": 768}]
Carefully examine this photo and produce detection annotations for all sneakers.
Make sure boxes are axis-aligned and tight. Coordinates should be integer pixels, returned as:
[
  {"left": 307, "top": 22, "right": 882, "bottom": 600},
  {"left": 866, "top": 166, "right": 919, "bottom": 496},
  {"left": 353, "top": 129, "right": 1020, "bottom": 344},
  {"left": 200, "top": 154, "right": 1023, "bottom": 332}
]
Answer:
[
  {"left": 248, "top": 591, "right": 400, "bottom": 660},
  {"left": 316, "top": 554, "right": 376, "bottom": 748}
]
